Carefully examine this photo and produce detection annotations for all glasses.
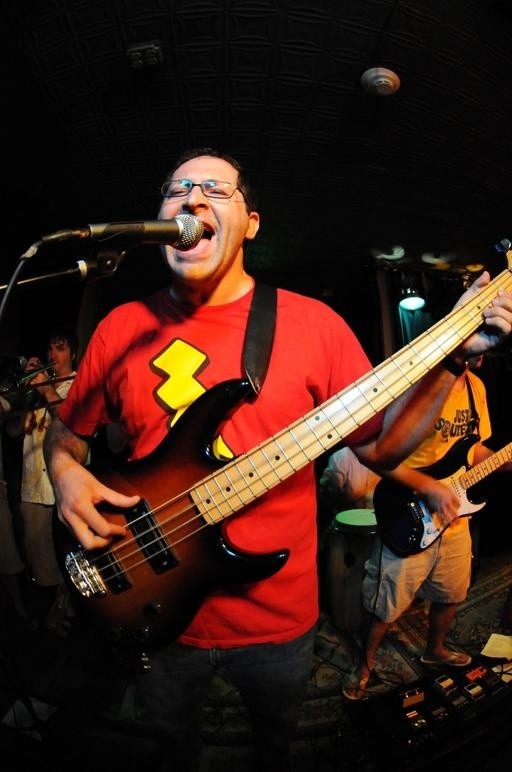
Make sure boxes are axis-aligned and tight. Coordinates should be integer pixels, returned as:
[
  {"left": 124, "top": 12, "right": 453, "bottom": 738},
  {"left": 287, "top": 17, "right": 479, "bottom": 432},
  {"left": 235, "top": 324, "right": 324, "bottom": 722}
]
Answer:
[{"left": 159, "top": 179, "right": 252, "bottom": 211}]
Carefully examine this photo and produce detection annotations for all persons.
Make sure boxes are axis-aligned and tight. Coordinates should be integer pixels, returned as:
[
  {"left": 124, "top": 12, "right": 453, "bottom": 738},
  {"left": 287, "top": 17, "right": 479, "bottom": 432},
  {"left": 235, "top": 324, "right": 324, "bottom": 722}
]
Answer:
[
  {"left": 313, "top": 446, "right": 383, "bottom": 615},
  {"left": 0, "top": 329, "right": 80, "bottom": 591},
  {"left": 42, "top": 143, "right": 510, "bottom": 771},
  {"left": 340, "top": 352, "right": 511, "bottom": 703}
]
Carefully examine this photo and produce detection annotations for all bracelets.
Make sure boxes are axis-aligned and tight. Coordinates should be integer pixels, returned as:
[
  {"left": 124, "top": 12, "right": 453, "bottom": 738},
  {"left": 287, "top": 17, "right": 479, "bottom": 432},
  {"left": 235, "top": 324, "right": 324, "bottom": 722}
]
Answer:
[{"left": 442, "top": 358, "right": 464, "bottom": 375}]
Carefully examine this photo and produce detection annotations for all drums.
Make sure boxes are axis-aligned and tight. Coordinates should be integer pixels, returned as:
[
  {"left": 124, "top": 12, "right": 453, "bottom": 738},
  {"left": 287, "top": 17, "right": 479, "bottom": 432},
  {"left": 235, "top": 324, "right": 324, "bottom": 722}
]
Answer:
[{"left": 331, "top": 505, "right": 376, "bottom": 544}]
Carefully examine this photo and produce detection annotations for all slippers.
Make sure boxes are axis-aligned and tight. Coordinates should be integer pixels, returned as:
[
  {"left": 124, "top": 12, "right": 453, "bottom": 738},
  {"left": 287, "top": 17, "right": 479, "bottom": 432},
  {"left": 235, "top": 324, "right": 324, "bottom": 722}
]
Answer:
[
  {"left": 343, "top": 666, "right": 370, "bottom": 701},
  {"left": 421, "top": 650, "right": 473, "bottom": 668}
]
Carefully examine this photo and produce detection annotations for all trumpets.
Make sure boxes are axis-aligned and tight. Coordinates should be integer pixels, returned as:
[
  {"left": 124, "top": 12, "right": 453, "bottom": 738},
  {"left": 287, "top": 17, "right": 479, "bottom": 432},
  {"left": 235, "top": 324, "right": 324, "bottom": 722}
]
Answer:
[{"left": 8, "top": 361, "right": 56, "bottom": 397}]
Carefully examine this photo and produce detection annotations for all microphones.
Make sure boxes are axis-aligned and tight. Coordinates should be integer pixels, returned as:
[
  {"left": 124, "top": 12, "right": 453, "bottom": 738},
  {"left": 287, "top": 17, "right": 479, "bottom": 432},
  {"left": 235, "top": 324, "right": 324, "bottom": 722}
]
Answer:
[{"left": 42, "top": 212, "right": 204, "bottom": 251}]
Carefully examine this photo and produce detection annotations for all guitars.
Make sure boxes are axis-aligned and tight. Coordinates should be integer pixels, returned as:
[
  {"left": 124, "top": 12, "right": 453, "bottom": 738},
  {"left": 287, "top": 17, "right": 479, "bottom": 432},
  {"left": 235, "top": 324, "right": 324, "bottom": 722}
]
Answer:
[
  {"left": 50, "top": 236, "right": 512, "bottom": 658},
  {"left": 374, "top": 434, "right": 512, "bottom": 556}
]
